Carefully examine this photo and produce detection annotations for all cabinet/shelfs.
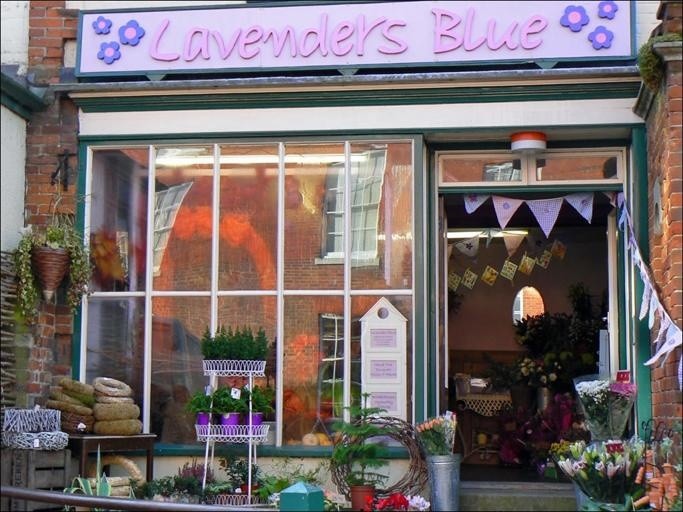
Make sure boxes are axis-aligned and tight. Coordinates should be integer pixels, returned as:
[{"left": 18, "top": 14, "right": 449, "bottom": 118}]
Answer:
[{"left": 194, "top": 359, "right": 266, "bottom": 505}]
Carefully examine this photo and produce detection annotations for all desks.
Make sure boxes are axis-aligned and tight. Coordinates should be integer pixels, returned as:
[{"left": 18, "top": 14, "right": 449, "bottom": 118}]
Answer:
[{"left": 66, "top": 432, "right": 158, "bottom": 482}]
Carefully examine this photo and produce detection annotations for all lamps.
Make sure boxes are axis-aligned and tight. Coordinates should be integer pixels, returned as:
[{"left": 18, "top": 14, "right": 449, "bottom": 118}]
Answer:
[
  {"left": 446, "top": 227, "right": 529, "bottom": 239},
  {"left": 511, "top": 131, "right": 547, "bottom": 154}
]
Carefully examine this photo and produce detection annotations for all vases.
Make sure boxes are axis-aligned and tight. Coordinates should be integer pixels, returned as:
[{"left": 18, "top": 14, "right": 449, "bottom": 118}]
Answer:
[
  {"left": 572, "top": 481, "right": 588, "bottom": 511},
  {"left": 536, "top": 385, "right": 550, "bottom": 410}
]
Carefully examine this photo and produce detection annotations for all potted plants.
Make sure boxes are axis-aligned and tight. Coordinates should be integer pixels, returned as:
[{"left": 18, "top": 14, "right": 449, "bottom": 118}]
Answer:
[
  {"left": 186, "top": 391, "right": 216, "bottom": 435},
  {"left": 219, "top": 453, "right": 259, "bottom": 503},
  {"left": 331, "top": 388, "right": 392, "bottom": 512},
  {"left": 214, "top": 382, "right": 250, "bottom": 436},
  {"left": 15, "top": 224, "right": 90, "bottom": 325},
  {"left": 201, "top": 325, "right": 270, "bottom": 371},
  {"left": 241, "top": 384, "right": 275, "bottom": 436}
]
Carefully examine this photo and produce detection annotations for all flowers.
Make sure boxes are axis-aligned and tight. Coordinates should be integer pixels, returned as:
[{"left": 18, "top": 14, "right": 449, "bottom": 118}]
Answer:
[
  {"left": 572, "top": 373, "right": 638, "bottom": 443},
  {"left": 415, "top": 409, "right": 457, "bottom": 455},
  {"left": 518, "top": 356, "right": 563, "bottom": 388},
  {"left": 550, "top": 434, "right": 645, "bottom": 504}
]
